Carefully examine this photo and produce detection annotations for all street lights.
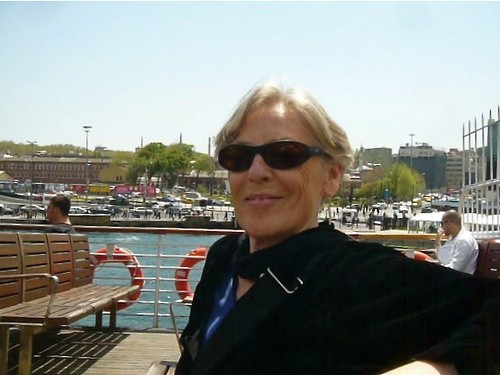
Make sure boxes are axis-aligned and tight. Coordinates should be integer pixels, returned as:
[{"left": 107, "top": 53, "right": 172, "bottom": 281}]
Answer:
[{"left": 84, "top": 125, "right": 93, "bottom": 202}]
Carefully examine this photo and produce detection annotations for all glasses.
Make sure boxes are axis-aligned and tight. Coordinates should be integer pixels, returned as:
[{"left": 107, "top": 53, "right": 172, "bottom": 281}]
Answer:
[
  {"left": 441, "top": 220, "right": 447, "bottom": 227},
  {"left": 217, "top": 140, "right": 323, "bottom": 174}
]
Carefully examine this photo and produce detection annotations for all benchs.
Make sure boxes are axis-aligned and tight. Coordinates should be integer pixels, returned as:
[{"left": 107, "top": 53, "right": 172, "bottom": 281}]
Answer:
[{"left": 0, "top": 232, "right": 141, "bottom": 375}]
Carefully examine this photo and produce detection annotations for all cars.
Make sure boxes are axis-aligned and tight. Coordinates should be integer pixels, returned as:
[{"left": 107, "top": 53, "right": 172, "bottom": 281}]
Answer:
[
  {"left": 372, "top": 202, "right": 388, "bottom": 210},
  {"left": 392, "top": 190, "right": 459, "bottom": 214},
  {"left": 345, "top": 203, "right": 360, "bottom": 209},
  {"left": 0, "top": 183, "right": 232, "bottom": 216}
]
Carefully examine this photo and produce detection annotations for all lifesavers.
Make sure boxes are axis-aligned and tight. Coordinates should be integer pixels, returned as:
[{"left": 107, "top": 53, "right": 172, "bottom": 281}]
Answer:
[
  {"left": 400, "top": 250, "right": 440, "bottom": 266},
  {"left": 88, "top": 246, "right": 145, "bottom": 312},
  {"left": 174, "top": 245, "right": 211, "bottom": 308}
]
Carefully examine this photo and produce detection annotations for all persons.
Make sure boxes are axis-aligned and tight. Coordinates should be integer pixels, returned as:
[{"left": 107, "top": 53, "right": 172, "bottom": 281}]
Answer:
[
  {"left": 10, "top": 197, "right": 229, "bottom": 282},
  {"left": 176, "top": 83, "right": 500, "bottom": 375}
]
